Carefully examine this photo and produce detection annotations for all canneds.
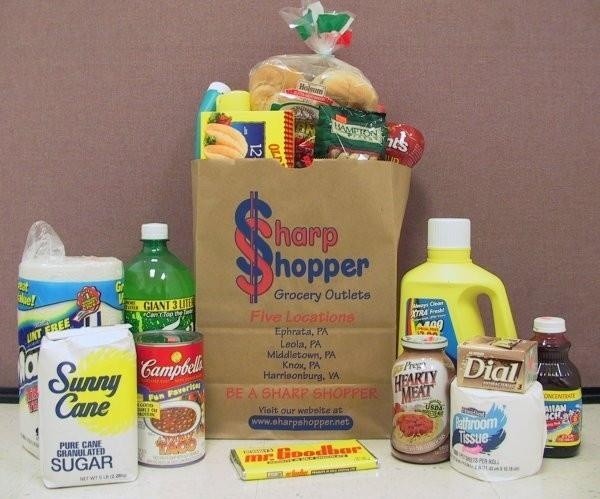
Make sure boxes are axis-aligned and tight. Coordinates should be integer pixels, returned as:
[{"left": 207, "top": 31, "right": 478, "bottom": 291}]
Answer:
[{"left": 135, "top": 331, "right": 207, "bottom": 469}]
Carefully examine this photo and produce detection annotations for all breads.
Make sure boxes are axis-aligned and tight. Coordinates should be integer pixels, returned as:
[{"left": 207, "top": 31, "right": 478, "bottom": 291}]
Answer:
[
  {"left": 200, "top": 146, "right": 241, "bottom": 161},
  {"left": 248, "top": 61, "right": 373, "bottom": 111},
  {"left": 205, "top": 123, "right": 249, "bottom": 157}
]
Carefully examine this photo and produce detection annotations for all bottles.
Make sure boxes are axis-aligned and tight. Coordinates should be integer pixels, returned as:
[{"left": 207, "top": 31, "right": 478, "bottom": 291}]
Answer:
[
  {"left": 390, "top": 335, "right": 456, "bottom": 465},
  {"left": 528, "top": 316, "right": 583, "bottom": 457},
  {"left": 123, "top": 222, "right": 195, "bottom": 331}
]
